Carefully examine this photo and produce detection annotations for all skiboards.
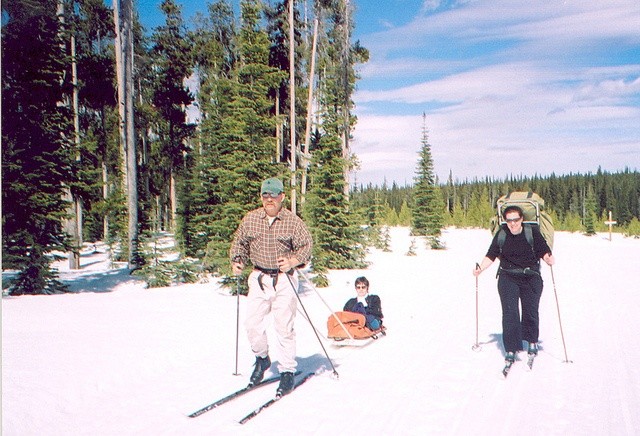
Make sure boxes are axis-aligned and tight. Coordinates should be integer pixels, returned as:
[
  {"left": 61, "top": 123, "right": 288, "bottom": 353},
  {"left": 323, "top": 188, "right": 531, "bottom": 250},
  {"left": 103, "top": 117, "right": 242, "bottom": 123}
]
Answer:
[
  {"left": 187, "top": 371, "right": 315, "bottom": 424},
  {"left": 503, "top": 346, "right": 536, "bottom": 378}
]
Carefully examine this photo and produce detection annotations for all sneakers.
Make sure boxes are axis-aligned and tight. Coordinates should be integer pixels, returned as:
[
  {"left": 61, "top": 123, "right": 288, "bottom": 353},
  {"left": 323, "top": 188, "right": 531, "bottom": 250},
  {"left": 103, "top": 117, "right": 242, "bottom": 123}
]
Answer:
[
  {"left": 506, "top": 352, "right": 514, "bottom": 361},
  {"left": 277, "top": 372, "right": 295, "bottom": 396},
  {"left": 251, "top": 355, "right": 271, "bottom": 382},
  {"left": 528, "top": 342, "right": 537, "bottom": 354}
]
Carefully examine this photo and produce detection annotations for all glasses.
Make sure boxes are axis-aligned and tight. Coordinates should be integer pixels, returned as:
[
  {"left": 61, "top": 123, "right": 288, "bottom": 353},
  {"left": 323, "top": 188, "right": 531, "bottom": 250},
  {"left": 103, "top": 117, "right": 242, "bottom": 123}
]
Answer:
[
  {"left": 357, "top": 286, "right": 367, "bottom": 289},
  {"left": 262, "top": 194, "right": 278, "bottom": 198},
  {"left": 505, "top": 218, "right": 521, "bottom": 223}
]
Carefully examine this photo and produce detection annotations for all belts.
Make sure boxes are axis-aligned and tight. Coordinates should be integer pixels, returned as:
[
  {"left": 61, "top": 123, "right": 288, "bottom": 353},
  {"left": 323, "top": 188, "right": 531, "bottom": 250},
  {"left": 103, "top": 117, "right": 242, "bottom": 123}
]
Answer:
[{"left": 255, "top": 265, "right": 282, "bottom": 274}]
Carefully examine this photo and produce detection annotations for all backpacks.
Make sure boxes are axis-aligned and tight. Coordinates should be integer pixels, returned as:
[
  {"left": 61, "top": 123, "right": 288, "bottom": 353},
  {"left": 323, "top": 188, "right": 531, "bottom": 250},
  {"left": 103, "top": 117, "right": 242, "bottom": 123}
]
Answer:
[{"left": 491, "top": 191, "right": 554, "bottom": 275}]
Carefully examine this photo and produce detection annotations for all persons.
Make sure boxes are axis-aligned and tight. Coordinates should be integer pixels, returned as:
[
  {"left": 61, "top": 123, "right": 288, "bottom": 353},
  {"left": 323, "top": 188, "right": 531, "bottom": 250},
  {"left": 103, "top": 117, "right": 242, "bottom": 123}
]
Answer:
[
  {"left": 473, "top": 203, "right": 551, "bottom": 362},
  {"left": 229, "top": 178, "right": 311, "bottom": 398},
  {"left": 343, "top": 275, "right": 384, "bottom": 333}
]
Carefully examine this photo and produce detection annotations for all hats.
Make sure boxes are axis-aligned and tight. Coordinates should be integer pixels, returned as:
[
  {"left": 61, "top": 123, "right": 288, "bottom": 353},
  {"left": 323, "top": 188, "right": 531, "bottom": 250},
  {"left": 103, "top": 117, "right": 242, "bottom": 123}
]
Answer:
[{"left": 261, "top": 178, "right": 283, "bottom": 194}]
